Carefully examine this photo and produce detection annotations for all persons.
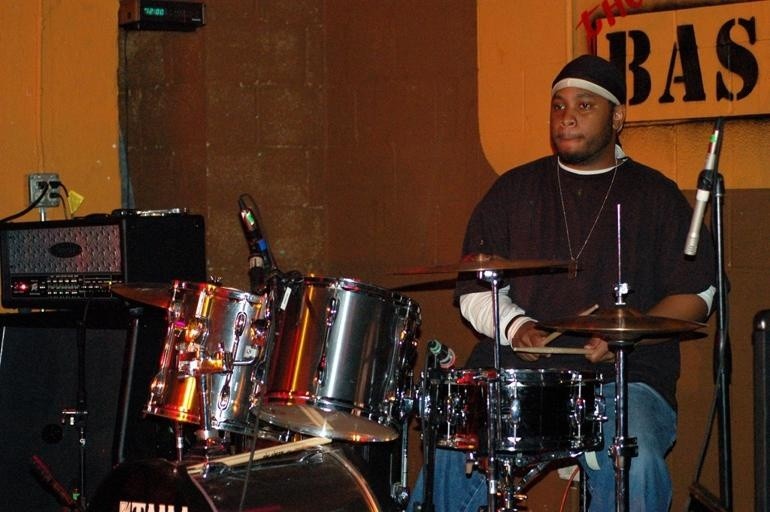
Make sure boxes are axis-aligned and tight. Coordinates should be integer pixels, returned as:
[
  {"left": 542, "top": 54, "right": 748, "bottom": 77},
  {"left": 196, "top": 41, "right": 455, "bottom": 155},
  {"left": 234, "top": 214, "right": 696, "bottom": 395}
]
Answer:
[{"left": 399, "top": 52, "right": 732, "bottom": 512}]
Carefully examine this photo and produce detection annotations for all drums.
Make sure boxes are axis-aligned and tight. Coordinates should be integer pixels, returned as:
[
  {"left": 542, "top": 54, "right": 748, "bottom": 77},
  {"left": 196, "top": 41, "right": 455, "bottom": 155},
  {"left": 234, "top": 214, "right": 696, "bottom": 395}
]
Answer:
[
  {"left": 252, "top": 278, "right": 423, "bottom": 444},
  {"left": 145, "top": 278, "right": 300, "bottom": 442},
  {"left": 422, "top": 369, "right": 608, "bottom": 451},
  {"left": 85, "top": 436, "right": 402, "bottom": 512}
]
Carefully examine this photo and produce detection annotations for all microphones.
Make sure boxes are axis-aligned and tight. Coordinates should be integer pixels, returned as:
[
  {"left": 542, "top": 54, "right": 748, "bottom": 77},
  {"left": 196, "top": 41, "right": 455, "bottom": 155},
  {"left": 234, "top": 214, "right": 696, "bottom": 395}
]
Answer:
[
  {"left": 430, "top": 339, "right": 458, "bottom": 368},
  {"left": 684, "top": 121, "right": 725, "bottom": 257},
  {"left": 241, "top": 207, "right": 269, "bottom": 267}
]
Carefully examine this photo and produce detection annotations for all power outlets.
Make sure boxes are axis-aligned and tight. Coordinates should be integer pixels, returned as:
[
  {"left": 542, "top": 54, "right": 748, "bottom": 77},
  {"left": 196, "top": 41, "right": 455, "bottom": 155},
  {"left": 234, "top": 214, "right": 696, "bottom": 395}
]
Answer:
[{"left": 26, "top": 171, "right": 65, "bottom": 210}]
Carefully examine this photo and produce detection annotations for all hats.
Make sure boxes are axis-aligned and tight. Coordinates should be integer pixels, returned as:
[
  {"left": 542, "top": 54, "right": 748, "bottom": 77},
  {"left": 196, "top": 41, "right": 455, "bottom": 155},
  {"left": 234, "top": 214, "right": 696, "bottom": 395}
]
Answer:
[{"left": 551, "top": 55, "right": 626, "bottom": 105}]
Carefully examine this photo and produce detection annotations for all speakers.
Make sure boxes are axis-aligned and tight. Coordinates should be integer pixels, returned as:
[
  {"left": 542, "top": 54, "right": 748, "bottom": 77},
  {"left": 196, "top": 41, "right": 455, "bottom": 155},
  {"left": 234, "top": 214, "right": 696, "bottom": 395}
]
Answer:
[{"left": 0, "top": 310, "right": 161, "bottom": 512}]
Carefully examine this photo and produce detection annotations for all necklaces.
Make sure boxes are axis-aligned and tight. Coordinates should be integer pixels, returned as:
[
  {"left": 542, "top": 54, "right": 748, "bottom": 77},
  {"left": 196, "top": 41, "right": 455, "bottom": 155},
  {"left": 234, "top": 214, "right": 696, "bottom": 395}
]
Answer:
[{"left": 556, "top": 154, "right": 619, "bottom": 279}]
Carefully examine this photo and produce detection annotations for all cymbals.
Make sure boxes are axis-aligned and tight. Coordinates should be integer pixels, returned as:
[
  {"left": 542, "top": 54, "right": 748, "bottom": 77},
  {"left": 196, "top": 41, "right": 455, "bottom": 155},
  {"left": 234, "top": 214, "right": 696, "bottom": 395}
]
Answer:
[
  {"left": 392, "top": 254, "right": 573, "bottom": 275},
  {"left": 535, "top": 308, "right": 706, "bottom": 333}
]
